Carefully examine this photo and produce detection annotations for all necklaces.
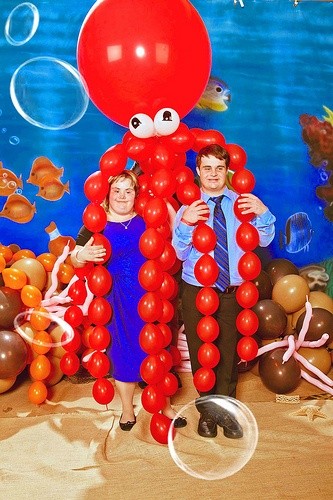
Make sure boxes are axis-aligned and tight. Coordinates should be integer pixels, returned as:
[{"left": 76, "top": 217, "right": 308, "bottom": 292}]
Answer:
[{"left": 109, "top": 212, "right": 132, "bottom": 230}]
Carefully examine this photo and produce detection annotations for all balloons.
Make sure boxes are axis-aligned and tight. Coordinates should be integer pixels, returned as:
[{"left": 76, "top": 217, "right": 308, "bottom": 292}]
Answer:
[{"left": 0, "top": 2, "right": 333, "bottom": 448}]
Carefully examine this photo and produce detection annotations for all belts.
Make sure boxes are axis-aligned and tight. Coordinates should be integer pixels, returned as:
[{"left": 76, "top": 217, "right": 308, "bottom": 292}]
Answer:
[{"left": 211, "top": 286, "right": 237, "bottom": 294}]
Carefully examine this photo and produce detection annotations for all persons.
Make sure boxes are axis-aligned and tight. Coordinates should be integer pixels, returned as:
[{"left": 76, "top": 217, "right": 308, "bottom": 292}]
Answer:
[
  {"left": 175, "top": 144, "right": 277, "bottom": 438},
  {"left": 71, "top": 170, "right": 185, "bottom": 428}
]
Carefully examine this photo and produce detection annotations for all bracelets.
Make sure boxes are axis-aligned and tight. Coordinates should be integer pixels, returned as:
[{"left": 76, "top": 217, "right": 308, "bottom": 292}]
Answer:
[{"left": 181, "top": 218, "right": 198, "bottom": 227}]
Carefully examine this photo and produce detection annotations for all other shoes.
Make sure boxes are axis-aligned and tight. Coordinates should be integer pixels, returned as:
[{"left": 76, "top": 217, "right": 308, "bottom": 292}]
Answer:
[
  {"left": 170, "top": 417, "right": 186, "bottom": 428},
  {"left": 223, "top": 420, "right": 243, "bottom": 438},
  {"left": 198, "top": 416, "right": 216, "bottom": 437},
  {"left": 119, "top": 413, "right": 137, "bottom": 432}
]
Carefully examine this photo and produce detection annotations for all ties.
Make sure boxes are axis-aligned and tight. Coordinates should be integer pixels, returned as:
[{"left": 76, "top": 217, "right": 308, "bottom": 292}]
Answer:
[{"left": 209, "top": 195, "right": 230, "bottom": 292}]
